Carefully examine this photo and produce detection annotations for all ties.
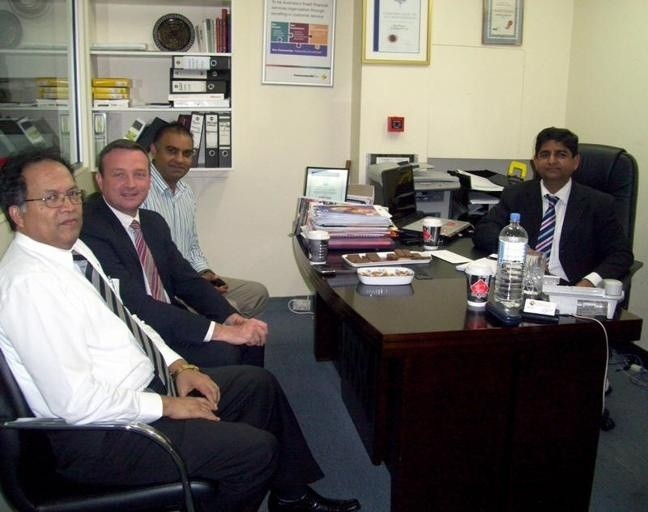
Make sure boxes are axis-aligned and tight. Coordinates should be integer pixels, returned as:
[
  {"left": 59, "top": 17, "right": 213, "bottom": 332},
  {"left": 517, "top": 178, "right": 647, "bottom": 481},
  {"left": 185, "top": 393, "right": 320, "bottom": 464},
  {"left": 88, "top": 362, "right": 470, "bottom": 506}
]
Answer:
[
  {"left": 71, "top": 250, "right": 177, "bottom": 397},
  {"left": 534, "top": 194, "right": 559, "bottom": 274},
  {"left": 131, "top": 220, "right": 166, "bottom": 302}
]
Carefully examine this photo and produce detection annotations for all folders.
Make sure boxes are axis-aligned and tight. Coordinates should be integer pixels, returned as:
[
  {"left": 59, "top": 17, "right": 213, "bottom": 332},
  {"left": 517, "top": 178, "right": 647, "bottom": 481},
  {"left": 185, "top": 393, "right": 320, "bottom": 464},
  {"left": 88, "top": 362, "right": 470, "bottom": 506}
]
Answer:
[
  {"left": 170, "top": 55, "right": 229, "bottom": 94},
  {"left": 190, "top": 111, "right": 231, "bottom": 168}
]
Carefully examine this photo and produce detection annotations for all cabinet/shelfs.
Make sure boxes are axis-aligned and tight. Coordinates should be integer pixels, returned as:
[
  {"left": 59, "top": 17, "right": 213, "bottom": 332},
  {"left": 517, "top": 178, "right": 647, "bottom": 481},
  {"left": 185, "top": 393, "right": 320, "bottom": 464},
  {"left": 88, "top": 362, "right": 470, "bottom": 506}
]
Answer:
[
  {"left": 73, "top": 1, "right": 237, "bottom": 173},
  {"left": 0, "top": 1, "right": 80, "bottom": 175}
]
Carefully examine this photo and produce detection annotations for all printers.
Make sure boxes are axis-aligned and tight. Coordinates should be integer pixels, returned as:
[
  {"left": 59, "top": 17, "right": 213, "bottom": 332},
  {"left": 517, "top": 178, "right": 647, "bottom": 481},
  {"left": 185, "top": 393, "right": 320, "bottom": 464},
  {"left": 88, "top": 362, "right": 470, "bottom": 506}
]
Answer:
[{"left": 367, "top": 161, "right": 461, "bottom": 219}]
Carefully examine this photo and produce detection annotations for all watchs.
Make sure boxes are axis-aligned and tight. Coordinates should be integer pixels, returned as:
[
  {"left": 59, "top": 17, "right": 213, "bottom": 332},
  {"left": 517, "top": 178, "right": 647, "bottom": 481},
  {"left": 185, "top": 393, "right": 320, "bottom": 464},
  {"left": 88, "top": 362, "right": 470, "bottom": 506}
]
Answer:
[{"left": 174, "top": 364, "right": 200, "bottom": 377}]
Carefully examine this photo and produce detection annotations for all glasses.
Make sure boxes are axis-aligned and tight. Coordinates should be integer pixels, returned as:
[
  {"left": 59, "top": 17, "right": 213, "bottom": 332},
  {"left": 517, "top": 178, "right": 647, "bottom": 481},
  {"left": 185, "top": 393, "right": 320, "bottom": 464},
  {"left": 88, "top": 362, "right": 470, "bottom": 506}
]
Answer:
[{"left": 24, "top": 190, "right": 86, "bottom": 208}]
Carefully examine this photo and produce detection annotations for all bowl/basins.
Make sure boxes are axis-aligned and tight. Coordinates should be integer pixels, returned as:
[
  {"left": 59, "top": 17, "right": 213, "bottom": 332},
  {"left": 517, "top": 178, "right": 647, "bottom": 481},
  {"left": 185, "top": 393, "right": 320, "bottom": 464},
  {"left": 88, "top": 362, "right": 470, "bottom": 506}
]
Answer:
[
  {"left": 354, "top": 267, "right": 415, "bottom": 286},
  {"left": 358, "top": 281, "right": 415, "bottom": 298}
]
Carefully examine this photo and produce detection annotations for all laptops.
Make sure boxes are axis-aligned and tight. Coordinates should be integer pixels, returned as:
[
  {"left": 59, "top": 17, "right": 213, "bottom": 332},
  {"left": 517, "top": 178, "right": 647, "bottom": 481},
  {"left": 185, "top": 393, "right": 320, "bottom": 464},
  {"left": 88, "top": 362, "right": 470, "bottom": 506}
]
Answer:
[{"left": 381, "top": 166, "right": 470, "bottom": 240}]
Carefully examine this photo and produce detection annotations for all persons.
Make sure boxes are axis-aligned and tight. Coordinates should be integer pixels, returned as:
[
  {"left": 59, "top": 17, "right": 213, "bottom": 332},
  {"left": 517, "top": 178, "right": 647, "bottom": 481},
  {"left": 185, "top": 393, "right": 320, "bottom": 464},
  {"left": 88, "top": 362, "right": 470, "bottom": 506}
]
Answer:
[
  {"left": 473, "top": 126, "right": 634, "bottom": 333},
  {"left": 139, "top": 126, "right": 268, "bottom": 326},
  {"left": 0, "top": 156, "right": 362, "bottom": 512},
  {"left": 77, "top": 140, "right": 267, "bottom": 368}
]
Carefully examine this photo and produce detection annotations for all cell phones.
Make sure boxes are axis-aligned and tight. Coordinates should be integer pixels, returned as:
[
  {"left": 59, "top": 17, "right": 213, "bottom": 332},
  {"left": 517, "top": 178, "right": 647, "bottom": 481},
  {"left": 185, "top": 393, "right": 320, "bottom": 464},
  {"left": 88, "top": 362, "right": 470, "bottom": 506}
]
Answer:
[
  {"left": 211, "top": 279, "right": 225, "bottom": 287},
  {"left": 313, "top": 264, "right": 336, "bottom": 274}
]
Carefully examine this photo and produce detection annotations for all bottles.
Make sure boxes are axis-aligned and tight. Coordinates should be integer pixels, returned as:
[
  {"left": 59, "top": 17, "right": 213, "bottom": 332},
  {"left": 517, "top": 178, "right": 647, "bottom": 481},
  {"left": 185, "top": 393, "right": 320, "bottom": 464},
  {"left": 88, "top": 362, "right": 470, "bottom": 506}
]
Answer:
[{"left": 493, "top": 211, "right": 529, "bottom": 302}]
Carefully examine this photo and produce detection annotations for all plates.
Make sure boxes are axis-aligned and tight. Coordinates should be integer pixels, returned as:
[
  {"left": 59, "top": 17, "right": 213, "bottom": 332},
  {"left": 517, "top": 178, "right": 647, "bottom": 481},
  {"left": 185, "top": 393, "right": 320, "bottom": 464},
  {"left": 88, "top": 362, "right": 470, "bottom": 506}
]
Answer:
[
  {"left": 153, "top": 13, "right": 196, "bottom": 51},
  {"left": 342, "top": 249, "right": 434, "bottom": 267},
  {"left": 7, "top": 1, "right": 53, "bottom": 19},
  {"left": 0, "top": 8, "right": 22, "bottom": 49}
]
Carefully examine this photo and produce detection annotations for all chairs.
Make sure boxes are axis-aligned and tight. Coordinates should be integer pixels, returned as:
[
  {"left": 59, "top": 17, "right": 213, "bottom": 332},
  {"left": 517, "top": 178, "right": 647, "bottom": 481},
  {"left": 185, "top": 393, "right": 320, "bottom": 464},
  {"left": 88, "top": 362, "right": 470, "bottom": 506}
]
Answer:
[
  {"left": 521, "top": 138, "right": 644, "bottom": 433},
  {"left": 0, "top": 346, "right": 222, "bottom": 510}
]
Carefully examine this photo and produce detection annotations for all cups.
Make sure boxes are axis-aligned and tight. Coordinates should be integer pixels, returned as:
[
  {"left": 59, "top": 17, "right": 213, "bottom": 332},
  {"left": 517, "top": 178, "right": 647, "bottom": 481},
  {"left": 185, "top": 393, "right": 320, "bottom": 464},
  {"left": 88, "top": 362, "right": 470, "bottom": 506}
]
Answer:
[
  {"left": 520, "top": 247, "right": 544, "bottom": 295},
  {"left": 423, "top": 219, "right": 441, "bottom": 250},
  {"left": 464, "top": 308, "right": 489, "bottom": 331},
  {"left": 463, "top": 265, "right": 493, "bottom": 310},
  {"left": 307, "top": 230, "right": 331, "bottom": 265}
]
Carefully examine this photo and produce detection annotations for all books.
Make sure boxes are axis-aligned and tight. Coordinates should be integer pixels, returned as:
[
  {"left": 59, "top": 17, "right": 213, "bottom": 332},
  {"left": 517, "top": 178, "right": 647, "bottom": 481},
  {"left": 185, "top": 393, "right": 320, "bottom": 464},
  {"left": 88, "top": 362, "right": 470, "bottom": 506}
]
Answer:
[
  {"left": 35, "top": 75, "right": 70, "bottom": 109},
  {"left": 293, "top": 195, "right": 395, "bottom": 251},
  {"left": 92, "top": 9, "right": 232, "bottom": 168}
]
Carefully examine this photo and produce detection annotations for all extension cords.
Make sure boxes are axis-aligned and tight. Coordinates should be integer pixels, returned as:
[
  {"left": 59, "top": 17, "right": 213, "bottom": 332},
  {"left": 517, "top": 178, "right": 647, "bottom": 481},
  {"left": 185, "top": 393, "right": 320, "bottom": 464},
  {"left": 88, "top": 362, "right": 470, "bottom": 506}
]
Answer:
[{"left": 629, "top": 363, "right": 642, "bottom": 371}]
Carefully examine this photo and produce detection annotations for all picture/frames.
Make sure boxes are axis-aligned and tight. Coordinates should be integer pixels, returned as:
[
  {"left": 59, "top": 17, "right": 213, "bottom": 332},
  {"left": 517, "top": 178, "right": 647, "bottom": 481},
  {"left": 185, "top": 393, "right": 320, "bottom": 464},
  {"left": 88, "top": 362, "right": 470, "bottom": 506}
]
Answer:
[
  {"left": 482, "top": 0, "right": 524, "bottom": 47},
  {"left": 358, "top": 1, "right": 432, "bottom": 67},
  {"left": 303, "top": 165, "right": 351, "bottom": 203},
  {"left": 259, "top": 1, "right": 338, "bottom": 89}
]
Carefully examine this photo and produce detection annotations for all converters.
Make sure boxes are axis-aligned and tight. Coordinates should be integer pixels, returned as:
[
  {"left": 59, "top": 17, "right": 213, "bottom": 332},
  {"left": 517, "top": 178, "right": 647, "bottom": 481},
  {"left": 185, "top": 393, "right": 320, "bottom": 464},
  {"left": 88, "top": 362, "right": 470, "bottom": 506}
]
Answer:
[
  {"left": 519, "top": 309, "right": 560, "bottom": 322},
  {"left": 292, "top": 299, "right": 311, "bottom": 311}
]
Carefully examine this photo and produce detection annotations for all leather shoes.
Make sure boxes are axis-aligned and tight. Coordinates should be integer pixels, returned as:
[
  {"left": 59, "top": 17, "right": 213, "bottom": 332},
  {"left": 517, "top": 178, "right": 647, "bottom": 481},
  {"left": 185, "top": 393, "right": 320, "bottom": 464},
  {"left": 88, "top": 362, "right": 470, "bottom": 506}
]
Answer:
[{"left": 268, "top": 487, "right": 360, "bottom": 512}]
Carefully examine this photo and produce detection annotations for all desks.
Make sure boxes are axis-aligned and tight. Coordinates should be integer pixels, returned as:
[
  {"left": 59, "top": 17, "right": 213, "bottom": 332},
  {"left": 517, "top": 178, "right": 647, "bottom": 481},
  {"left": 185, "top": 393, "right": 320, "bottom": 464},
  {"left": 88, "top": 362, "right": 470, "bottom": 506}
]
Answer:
[{"left": 291, "top": 205, "right": 643, "bottom": 512}]
